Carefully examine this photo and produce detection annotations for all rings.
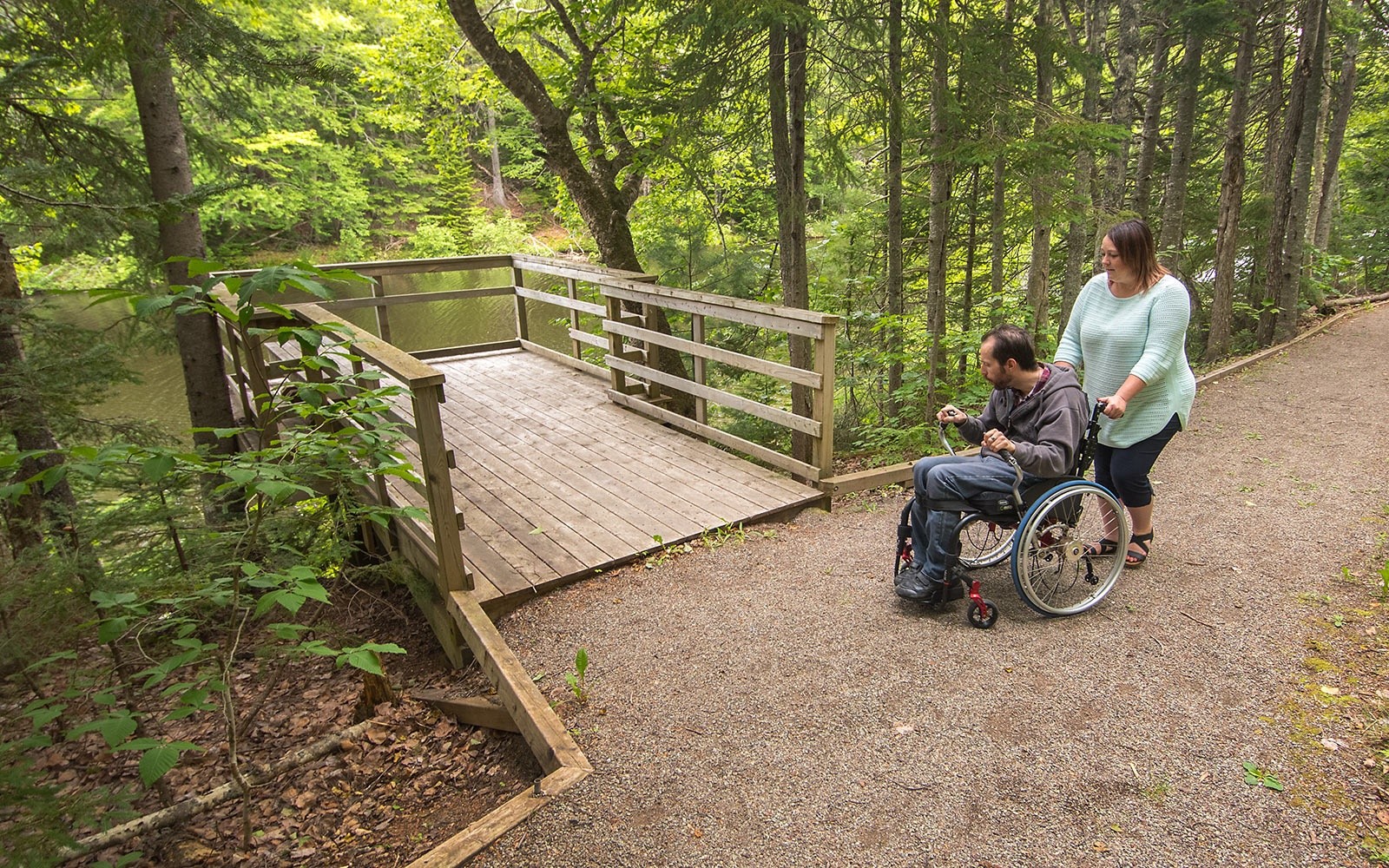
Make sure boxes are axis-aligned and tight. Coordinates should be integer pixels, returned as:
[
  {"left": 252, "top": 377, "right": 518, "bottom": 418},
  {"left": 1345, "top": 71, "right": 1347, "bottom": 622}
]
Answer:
[{"left": 991, "top": 435, "right": 997, "bottom": 443}]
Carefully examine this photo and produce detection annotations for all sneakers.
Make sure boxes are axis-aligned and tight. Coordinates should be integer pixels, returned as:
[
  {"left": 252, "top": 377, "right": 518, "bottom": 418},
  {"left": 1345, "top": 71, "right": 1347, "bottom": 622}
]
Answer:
[
  {"left": 895, "top": 571, "right": 963, "bottom": 601},
  {"left": 895, "top": 561, "right": 923, "bottom": 584}
]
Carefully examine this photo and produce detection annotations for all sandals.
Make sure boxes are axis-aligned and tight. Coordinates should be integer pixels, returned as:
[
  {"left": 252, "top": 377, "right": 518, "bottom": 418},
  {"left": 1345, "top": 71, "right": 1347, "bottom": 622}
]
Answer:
[
  {"left": 1125, "top": 527, "right": 1154, "bottom": 567},
  {"left": 1082, "top": 538, "right": 1118, "bottom": 557}
]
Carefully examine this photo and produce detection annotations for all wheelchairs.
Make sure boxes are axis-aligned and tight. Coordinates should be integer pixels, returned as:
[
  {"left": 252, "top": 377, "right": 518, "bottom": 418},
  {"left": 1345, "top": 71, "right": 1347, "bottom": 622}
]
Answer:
[{"left": 893, "top": 399, "right": 1130, "bottom": 630}]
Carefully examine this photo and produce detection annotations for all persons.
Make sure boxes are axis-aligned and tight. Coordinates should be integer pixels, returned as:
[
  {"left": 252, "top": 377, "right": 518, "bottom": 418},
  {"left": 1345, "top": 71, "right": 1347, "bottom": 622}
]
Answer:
[
  {"left": 1054, "top": 220, "right": 1197, "bottom": 567},
  {"left": 894, "top": 324, "right": 1090, "bottom": 601}
]
